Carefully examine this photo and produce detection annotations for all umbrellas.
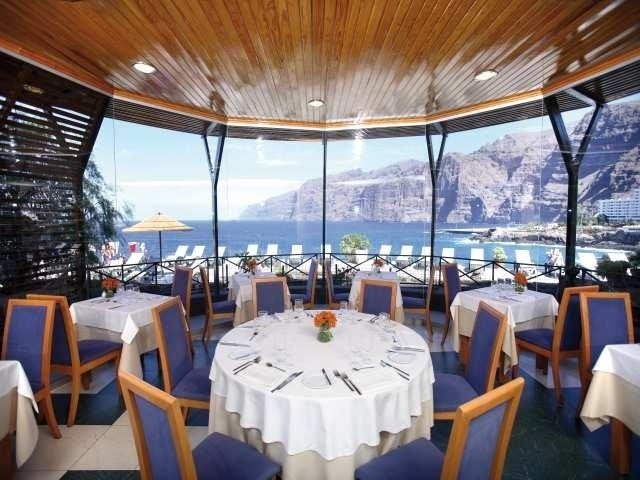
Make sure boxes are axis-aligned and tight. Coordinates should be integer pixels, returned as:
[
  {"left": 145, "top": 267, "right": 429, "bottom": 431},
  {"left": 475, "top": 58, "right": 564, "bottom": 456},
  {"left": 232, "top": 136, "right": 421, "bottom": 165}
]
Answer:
[{"left": 121, "top": 213, "right": 195, "bottom": 262}]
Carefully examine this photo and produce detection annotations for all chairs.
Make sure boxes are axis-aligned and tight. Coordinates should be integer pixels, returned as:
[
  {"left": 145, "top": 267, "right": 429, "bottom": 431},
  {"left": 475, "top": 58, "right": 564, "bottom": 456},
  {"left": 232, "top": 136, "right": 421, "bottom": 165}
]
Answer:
[
  {"left": 251, "top": 277, "right": 288, "bottom": 319},
  {"left": 170, "top": 267, "right": 194, "bottom": 324},
  {"left": 355, "top": 376, "right": 526, "bottom": 479},
  {"left": 118, "top": 370, "right": 284, "bottom": 479},
  {"left": 401, "top": 266, "right": 435, "bottom": 342},
  {"left": 151, "top": 298, "right": 210, "bottom": 426},
  {"left": 431, "top": 300, "right": 509, "bottom": 421},
  {"left": 324, "top": 261, "right": 350, "bottom": 310},
  {"left": 0, "top": 298, "right": 62, "bottom": 443},
  {"left": 358, "top": 280, "right": 398, "bottom": 322},
  {"left": 441, "top": 263, "right": 463, "bottom": 346},
  {"left": 26, "top": 292, "right": 123, "bottom": 428},
  {"left": 516, "top": 284, "right": 598, "bottom": 401},
  {"left": 579, "top": 291, "right": 640, "bottom": 475},
  {"left": 290, "top": 259, "right": 319, "bottom": 309},
  {"left": 198, "top": 270, "right": 236, "bottom": 342}
]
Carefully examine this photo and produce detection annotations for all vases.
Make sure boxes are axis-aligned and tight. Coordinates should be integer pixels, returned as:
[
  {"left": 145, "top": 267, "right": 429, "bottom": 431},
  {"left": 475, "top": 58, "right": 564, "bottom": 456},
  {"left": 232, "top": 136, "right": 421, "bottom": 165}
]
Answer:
[
  {"left": 317, "top": 324, "right": 333, "bottom": 342},
  {"left": 106, "top": 289, "right": 114, "bottom": 297},
  {"left": 516, "top": 284, "right": 526, "bottom": 292}
]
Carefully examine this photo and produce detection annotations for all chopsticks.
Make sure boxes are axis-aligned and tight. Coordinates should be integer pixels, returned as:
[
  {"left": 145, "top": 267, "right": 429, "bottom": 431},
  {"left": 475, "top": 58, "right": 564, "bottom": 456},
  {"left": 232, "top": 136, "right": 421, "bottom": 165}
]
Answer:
[{"left": 380, "top": 360, "right": 409, "bottom": 381}]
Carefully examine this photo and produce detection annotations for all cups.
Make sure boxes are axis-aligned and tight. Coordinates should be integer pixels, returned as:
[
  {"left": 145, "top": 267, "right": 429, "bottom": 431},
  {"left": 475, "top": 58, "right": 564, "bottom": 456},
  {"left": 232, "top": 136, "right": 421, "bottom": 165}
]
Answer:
[
  {"left": 349, "top": 302, "right": 357, "bottom": 316},
  {"left": 117, "top": 283, "right": 125, "bottom": 294},
  {"left": 339, "top": 301, "right": 349, "bottom": 315},
  {"left": 132, "top": 287, "right": 139, "bottom": 299},
  {"left": 125, "top": 284, "right": 134, "bottom": 300},
  {"left": 294, "top": 299, "right": 303, "bottom": 314},
  {"left": 295, "top": 298, "right": 304, "bottom": 317}
]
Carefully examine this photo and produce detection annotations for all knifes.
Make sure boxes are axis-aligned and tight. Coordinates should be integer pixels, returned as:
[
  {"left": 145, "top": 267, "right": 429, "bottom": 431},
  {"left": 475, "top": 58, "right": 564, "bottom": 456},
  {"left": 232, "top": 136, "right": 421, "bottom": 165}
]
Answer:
[
  {"left": 278, "top": 371, "right": 303, "bottom": 390},
  {"left": 322, "top": 368, "right": 332, "bottom": 386},
  {"left": 271, "top": 373, "right": 297, "bottom": 393}
]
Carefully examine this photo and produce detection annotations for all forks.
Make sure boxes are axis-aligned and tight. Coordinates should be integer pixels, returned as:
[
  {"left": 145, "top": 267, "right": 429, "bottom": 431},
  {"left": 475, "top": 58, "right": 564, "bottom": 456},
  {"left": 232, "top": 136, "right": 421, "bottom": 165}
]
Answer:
[
  {"left": 230, "top": 355, "right": 261, "bottom": 372},
  {"left": 333, "top": 369, "right": 354, "bottom": 390},
  {"left": 233, "top": 359, "right": 266, "bottom": 381},
  {"left": 340, "top": 372, "right": 362, "bottom": 396}
]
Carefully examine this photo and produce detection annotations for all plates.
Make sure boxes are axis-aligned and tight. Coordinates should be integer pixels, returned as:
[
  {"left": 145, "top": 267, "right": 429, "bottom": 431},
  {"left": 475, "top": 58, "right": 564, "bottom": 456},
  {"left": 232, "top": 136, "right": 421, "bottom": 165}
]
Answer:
[
  {"left": 230, "top": 350, "right": 255, "bottom": 359},
  {"left": 301, "top": 372, "right": 334, "bottom": 389},
  {"left": 388, "top": 352, "right": 414, "bottom": 363}
]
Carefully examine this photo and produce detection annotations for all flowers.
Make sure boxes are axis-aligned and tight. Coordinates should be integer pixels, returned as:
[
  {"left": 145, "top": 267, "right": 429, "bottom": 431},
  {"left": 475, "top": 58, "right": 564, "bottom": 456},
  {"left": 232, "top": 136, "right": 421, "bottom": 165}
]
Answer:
[
  {"left": 515, "top": 272, "right": 528, "bottom": 284},
  {"left": 313, "top": 311, "right": 337, "bottom": 328},
  {"left": 102, "top": 278, "right": 119, "bottom": 290}
]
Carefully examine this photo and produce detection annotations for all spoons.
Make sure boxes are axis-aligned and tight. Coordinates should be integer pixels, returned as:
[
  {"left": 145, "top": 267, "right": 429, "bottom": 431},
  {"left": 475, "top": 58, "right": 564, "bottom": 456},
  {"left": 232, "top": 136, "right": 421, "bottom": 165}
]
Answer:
[
  {"left": 248, "top": 330, "right": 259, "bottom": 341},
  {"left": 265, "top": 362, "right": 289, "bottom": 374},
  {"left": 353, "top": 365, "right": 375, "bottom": 371}
]
